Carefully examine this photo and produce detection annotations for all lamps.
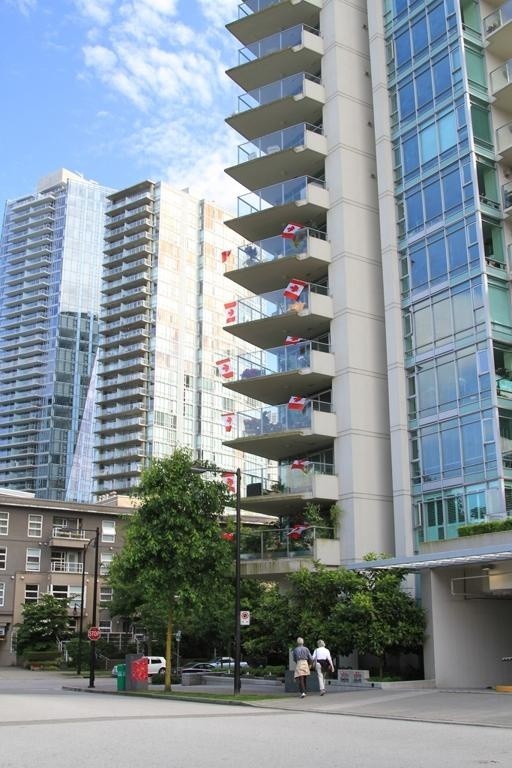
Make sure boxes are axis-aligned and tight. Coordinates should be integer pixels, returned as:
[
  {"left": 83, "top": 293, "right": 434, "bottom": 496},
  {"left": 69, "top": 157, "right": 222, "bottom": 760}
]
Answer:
[{"left": 480, "top": 564, "right": 495, "bottom": 571}]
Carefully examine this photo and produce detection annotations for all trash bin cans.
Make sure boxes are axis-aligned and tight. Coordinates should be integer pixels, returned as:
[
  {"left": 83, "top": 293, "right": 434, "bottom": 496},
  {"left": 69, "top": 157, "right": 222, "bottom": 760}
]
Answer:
[{"left": 117, "top": 664, "right": 126, "bottom": 689}]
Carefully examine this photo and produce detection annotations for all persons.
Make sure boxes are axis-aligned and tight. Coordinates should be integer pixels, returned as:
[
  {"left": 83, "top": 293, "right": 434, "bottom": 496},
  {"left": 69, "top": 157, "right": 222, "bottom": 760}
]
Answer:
[
  {"left": 290, "top": 636, "right": 313, "bottom": 696},
  {"left": 311, "top": 639, "right": 336, "bottom": 695}
]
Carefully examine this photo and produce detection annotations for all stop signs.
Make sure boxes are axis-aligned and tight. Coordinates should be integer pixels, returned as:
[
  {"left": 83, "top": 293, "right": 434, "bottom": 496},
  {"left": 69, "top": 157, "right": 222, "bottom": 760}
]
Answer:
[{"left": 88, "top": 627, "right": 101, "bottom": 641}]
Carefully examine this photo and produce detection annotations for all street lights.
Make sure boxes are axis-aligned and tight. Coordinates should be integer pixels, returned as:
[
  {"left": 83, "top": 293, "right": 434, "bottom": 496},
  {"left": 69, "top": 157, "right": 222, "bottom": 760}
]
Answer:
[
  {"left": 57, "top": 527, "right": 100, "bottom": 688},
  {"left": 190, "top": 467, "right": 240, "bottom": 695}
]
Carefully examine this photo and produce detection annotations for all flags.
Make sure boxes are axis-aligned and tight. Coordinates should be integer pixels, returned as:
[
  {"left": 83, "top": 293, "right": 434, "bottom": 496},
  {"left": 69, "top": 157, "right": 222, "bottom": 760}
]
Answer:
[
  {"left": 282, "top": 335, "right": 302, "bottom": 346},
  {"left": 283, "top": 279, "right": 306, "bottom": 301},
  {"left": 280, "top": 222, "right": 301, "bottom": 238},
  {"left": 285, "top": 525, "right": 308, "bottom": 540},
  {"left": 292, "top": 458, "right": 309, "bottom": 470},
  {"left": 221, "top": 473, "right": 238, "bottom": 495},
  {"left": 288, "top": 394, "right": 308, "bottom": 411},
  {"left": 221, "top": 412, "right": 236, "bottom": 433},
  {"left": 216, "top": 358, "right": 234, "bottom": 378},
  {"left": 221, "top": 250, "right": 231, "bottom": 263},
  {"left": 225, "top": 302, "right": 236, "bottom": 324}
]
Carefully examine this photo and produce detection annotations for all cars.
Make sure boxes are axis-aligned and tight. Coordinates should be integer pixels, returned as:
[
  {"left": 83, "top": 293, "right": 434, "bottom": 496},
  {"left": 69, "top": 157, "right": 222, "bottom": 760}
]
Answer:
[{"left": 112, "top": 656, "right": 249, "bottom": 677}]
